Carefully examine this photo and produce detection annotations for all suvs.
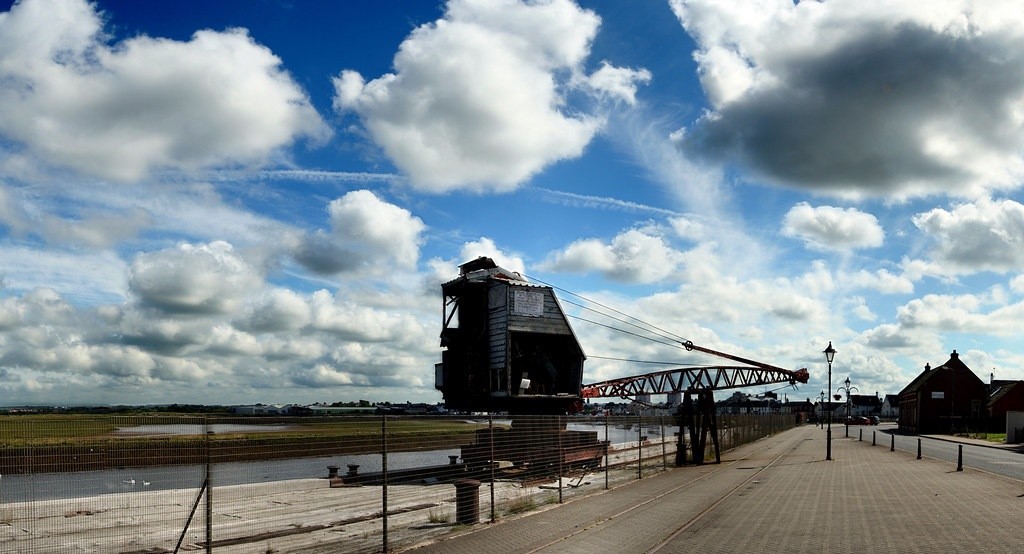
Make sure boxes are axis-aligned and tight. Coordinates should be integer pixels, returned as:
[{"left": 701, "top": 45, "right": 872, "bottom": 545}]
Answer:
[{"left": 865, "top": 416, "right": 879, "bottom": 426}]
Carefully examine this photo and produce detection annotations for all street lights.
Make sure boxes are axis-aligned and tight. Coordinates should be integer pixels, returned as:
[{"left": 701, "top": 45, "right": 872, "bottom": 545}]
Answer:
[
  {"left": 838, "top": 377, "right": 859, "bottom": 437},
  {"left": 823, "top": 341, "right": 838, "bottom": 460},
  {"left": 817, "top": 391, "right": 829, "bottom": 430}
]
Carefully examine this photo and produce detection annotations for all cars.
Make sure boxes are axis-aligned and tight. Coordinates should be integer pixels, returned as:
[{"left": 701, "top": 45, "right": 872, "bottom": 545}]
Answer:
[{"left": 845, "top": 416, "right": 870, "bottom": 425}]
